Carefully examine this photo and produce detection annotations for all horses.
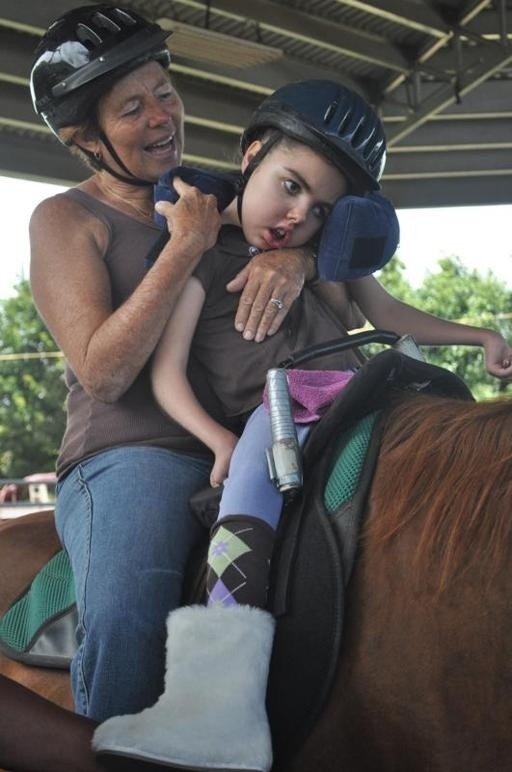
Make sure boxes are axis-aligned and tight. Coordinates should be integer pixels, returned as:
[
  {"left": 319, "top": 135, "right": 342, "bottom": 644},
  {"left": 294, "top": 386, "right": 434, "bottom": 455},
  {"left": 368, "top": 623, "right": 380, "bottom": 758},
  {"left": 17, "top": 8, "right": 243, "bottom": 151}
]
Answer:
[{"left": 0, "top": 397, "right": 512, "bottom": 772}]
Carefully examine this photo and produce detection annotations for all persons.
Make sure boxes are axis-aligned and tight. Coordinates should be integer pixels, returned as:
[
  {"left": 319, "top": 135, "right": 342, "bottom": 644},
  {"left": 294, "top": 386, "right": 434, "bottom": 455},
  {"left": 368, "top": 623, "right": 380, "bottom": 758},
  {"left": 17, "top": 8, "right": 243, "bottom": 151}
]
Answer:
[
  {"left": 89, "top": 79, "right": 512, "bottom": 772},
  {"left": 28, "top": 3, "right": 363, "bottom": 772}
]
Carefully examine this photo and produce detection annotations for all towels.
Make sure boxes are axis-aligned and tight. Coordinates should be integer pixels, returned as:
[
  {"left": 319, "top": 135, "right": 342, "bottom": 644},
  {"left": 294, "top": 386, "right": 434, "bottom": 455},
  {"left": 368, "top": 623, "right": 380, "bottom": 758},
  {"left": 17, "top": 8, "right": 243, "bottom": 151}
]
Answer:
[
  {"left": 267, "top": 369, "right": 357, "bottom": 422},
  {"left": 151, "top": 165, "right": 244, "bottom": 230}
]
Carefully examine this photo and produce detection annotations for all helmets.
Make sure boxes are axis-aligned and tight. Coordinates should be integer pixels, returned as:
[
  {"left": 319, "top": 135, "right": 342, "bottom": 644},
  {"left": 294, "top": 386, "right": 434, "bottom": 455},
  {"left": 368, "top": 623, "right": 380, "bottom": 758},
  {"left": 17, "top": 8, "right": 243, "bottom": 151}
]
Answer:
[
  {"left": 29, "top": 4, "right": 174, "bottom": 145},
  {"left": 241, "top": 79, "right": 388, "bottom": 197}
]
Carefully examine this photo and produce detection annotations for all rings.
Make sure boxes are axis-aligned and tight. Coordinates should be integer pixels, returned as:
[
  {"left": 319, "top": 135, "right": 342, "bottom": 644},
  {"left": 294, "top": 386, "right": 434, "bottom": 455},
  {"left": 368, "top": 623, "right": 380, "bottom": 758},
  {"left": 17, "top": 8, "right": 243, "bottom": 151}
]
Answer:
[{"left": 271, "top": 298, "right": 283, "bottom": 310}]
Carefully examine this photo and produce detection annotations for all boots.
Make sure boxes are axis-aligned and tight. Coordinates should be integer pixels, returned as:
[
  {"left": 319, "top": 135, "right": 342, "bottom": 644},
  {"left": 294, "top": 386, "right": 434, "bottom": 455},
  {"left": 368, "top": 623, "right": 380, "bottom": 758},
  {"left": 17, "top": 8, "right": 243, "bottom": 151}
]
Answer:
[{"left": 92, "top": 604, "right": 276, "bottom": 772}]
